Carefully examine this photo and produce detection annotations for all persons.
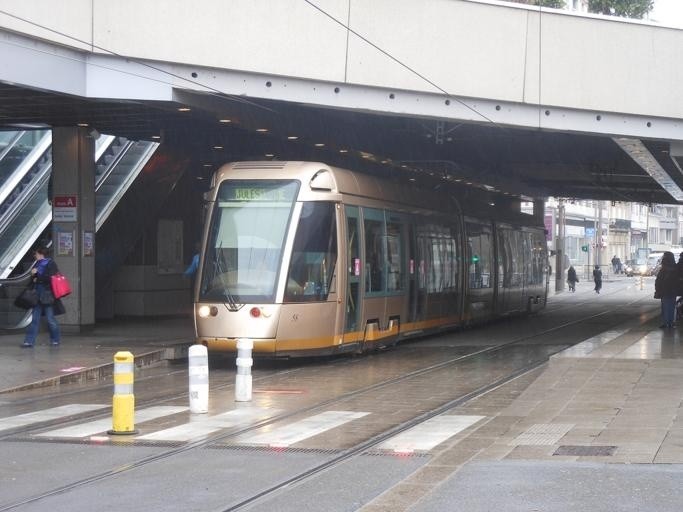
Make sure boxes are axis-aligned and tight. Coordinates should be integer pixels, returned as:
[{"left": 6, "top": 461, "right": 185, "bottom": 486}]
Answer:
[
  {"left": 611, "top": 255, "right": 617, "bottom": 274},
  {"left": 674, "top": 252, "right": 682, "bottom": 302},
  {"left": 653, "top": 252, "right": 679, "bottom": 329},
  {"left": 19, "top": 247, "right": 66, "bottom": 349},
  {"left": 591, "top": 265, "right": 603, "bottom": 294},
  {"left": 564, "top": 265, "right": 579, "bottom": 293},
  {"left": 180, "top": 238, "right": 212, "bottom": 299}
]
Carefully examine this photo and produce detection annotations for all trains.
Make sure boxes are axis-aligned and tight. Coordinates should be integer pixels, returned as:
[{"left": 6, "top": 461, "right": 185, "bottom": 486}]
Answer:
[{"left": 189, "top": 156, "right": 552, "bottom": 361}]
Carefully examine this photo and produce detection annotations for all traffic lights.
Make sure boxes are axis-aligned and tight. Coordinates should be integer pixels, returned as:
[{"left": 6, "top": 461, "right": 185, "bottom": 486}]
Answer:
[{"left": 582, "top": 246, "right": 588, "bottom": 252}]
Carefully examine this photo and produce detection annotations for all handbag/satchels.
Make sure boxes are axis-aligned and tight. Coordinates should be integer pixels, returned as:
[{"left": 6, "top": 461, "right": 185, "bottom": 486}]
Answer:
[
  {"left": 39, "top": 290, "right": 54, "bottom": 305},
  {"left": 50, "top": 272, "right": 72, "bottom": 299},
  {"left": 14, "top": 288, "right": 38, "bottom": 310}
]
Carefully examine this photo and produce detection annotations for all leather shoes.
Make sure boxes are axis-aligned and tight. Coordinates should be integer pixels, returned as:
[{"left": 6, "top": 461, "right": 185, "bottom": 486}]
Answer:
[{"left": 659, "top": 320, "right": 673, "bottom": 328}]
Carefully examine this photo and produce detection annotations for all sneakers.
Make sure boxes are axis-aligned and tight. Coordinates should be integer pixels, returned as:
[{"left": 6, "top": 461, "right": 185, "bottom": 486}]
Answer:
[
  {"left": 49, "top": 341, "right": 59, "bottom": 346},
  {"left": 21, "top": 343, "right": 32, "bottom": 347}
]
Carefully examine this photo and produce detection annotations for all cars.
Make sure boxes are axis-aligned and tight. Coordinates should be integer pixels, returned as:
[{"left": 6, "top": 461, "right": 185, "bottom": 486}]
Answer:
[{"left": 624, "top": 246, "right": 683, "bottom": 278}]
